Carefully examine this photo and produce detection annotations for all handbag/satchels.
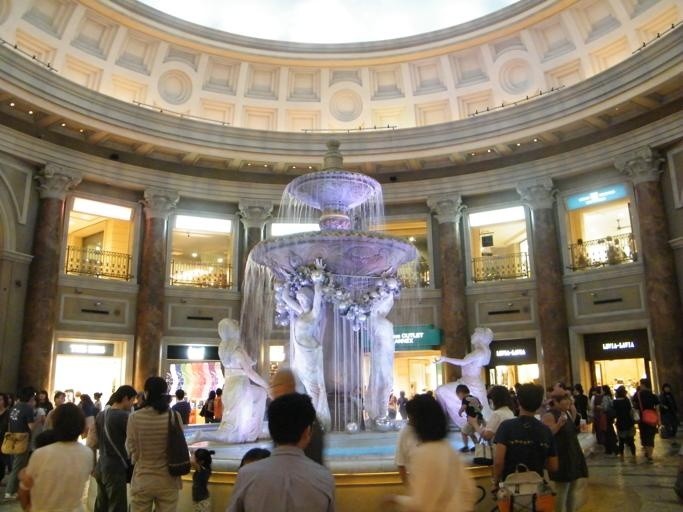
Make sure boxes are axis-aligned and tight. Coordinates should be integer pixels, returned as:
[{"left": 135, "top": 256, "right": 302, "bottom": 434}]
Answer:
[
  {"left": 200, "top": 405, "right": 208, "bottom": 416},
  {"left": 599, "top": 408, "right": 607, "bottom": 431},
  {"left": 497, "top": 463, "right": 558, "bottom": 512},
  {"left": 473, "top": 426, "right": 492, "bottom": 465},
  {"left": 641, "top": 409, "right": 657, "bottom": 425},
  {"left": 0, "top": 432, "right": 30, "bottom": 455},
  {"left": 628, "top": 399, "right": 640, "bottom": 421},
  {"left": 166, "top": 408, "right": 191, "bottom": 477}
]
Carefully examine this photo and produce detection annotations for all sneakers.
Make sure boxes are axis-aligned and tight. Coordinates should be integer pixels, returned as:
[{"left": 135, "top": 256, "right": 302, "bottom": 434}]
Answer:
[
  {"left": 4, "top": 493, "right": 16, "bottom": 499},
  {"left": 458, "top": 447, "right": 468, "bottom": 452},
  {"left": 470, "top": 447, "right": 475, "bottom": 452}
]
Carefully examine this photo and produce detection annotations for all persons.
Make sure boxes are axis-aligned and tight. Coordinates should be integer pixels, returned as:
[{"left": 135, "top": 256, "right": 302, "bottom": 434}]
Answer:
[
  {"left": 218, "top": 317, "right": 267, "bottom": 390},
  {"left": 281, "top": 258, "right": 331, "bottom": 432},
  {"left": 597, "top": 235, "right": 637, "bottom": 265},
  {"left": 0, "top": 371, "right": 683, "bottom": 512},
  {"left": 432, "top": 326, "right": 492, "bottom": 428},
  {"left": 575, "top": 239, "right": 587, "bottom": 267},
  {"left": 370, "top": 294, "right": 396, "bottom": 417}
]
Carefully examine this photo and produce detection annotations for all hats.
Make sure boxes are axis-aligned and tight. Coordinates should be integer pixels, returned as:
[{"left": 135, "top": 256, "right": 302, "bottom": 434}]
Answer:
[{"left": 614, "top": 386, "right": 627, "bottom": 393}]
